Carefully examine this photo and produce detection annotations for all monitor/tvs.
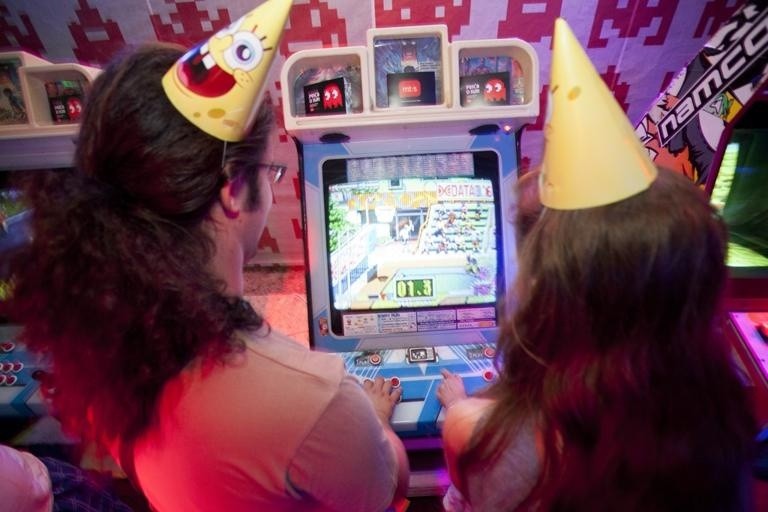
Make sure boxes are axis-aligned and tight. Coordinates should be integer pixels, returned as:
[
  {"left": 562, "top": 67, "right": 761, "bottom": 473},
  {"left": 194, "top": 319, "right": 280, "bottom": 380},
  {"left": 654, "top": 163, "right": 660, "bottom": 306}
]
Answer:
[{"left": 321, "top": 149, "right": 506, "bottom": 338}]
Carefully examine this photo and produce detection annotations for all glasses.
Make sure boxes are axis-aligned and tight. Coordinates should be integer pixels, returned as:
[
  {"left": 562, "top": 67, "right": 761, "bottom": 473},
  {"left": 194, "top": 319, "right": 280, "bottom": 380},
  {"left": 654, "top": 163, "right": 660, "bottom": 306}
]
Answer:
[{"left": 257, "top": 164, "right": 285, "bottom": 184}]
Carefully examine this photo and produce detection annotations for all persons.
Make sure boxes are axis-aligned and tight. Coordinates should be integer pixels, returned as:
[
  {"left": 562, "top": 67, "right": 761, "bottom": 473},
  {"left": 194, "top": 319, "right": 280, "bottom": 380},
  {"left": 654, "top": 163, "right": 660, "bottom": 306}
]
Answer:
[
  {"left": 437, "top": 167, "right": 767, "bottom": 512},
  {"left": 0, "top": 43, "right": 410, "bottom": 512}
]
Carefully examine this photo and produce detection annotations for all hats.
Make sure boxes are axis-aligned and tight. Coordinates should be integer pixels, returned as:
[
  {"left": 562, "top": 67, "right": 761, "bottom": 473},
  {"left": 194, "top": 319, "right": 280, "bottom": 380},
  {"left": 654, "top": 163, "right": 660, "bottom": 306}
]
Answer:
[
  {"left": 161, "top": 0, "right": 292, "bottom": 142},
  {"left": 540, "top": 18, "right": 658, "bottom": 210}
]
